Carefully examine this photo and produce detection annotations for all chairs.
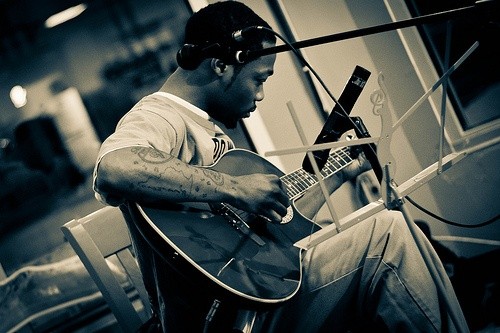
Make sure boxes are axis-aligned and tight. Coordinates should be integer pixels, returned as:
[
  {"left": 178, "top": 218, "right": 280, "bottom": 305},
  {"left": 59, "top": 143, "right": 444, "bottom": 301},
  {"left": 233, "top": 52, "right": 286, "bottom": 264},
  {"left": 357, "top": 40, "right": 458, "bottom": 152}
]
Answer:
[{"left": 61, "top": 205, "right": 165, "bottom": 333}]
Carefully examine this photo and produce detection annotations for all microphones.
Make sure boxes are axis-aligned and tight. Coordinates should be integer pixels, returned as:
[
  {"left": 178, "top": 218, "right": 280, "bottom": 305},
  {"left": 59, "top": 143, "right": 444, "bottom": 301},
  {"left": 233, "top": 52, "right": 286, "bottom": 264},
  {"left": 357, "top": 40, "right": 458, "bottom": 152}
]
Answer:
[
  {"left": 301, "top": 65, "right": 371, "bottom": 175},
  {"left": 177, "top": 25, "right": 260, "bottom": 71}
]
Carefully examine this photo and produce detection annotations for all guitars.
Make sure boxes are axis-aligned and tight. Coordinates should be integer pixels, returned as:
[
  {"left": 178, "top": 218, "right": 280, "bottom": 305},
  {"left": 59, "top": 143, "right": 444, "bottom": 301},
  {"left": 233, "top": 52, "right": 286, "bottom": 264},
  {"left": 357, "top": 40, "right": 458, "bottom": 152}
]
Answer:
[{"left": 126, "top": 128, "right": 371, "bottom": 310}]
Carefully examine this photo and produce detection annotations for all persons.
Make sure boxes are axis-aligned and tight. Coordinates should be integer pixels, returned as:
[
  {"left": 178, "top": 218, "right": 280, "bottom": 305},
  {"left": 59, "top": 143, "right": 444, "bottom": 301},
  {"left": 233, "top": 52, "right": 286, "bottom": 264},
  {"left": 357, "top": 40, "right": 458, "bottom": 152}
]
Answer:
[{"left": 92, "top": 0, "right": 479, "bottom": 333}]
